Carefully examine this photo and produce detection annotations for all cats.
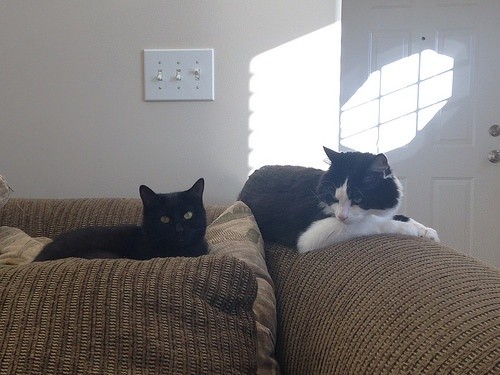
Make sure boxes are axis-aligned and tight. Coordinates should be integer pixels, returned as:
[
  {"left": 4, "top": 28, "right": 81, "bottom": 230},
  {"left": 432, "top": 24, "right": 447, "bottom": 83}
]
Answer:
[
  {"left": 235, "top": 146, "right": 441, "bottom": 253},
  {"left": 31, "top": 177, "right": 207, "bottom": 262}
]
